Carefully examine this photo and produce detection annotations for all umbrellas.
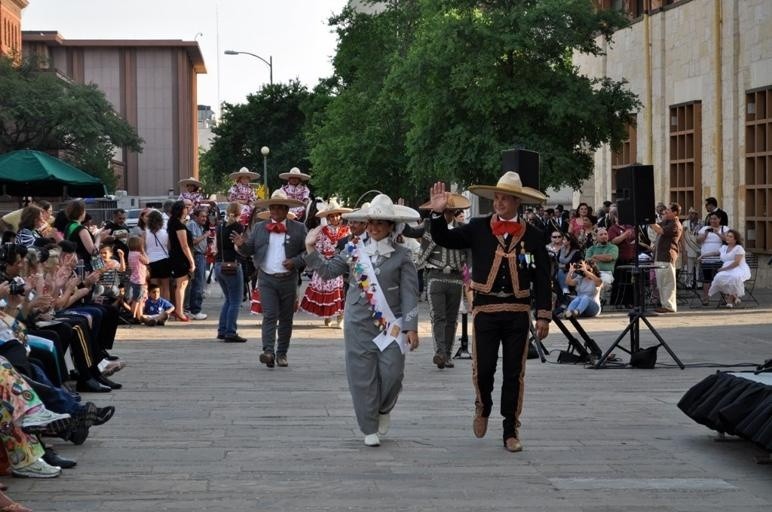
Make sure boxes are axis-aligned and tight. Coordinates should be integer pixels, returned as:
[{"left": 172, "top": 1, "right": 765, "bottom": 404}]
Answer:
[{"left": 0, "top": 145, "right": 105, "bottom": 208}]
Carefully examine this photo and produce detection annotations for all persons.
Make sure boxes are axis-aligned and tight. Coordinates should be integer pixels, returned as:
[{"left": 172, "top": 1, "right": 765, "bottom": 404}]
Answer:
[
  {"left": 304, "top": 193, "right": 421, "bottom": 448},
  {"left": 429, "top": 168, "right": 553, "bottom": 453},
  {"left": 522, "top": 196, "right": 751, "bottom": 320},
  {"left": 228, "top": 188, "right": 308, "bottom": 369},
  {"left": 277, "top": 167, "right": 313, "bottom": 220},
  {"left": 2, "top": 166, "right": 262, "bottom": 478},
  {"left": 298, "top": 201, "right": 354, "bottom": 329},
  {"left": 250, "top": 210, "right": 299, "bottom": 317},
  {"left": 399, "top": 192, "right": 472, "bottom": 369},
  {"left": 452, "top": 210, "right": 466, "bottom": 228}
]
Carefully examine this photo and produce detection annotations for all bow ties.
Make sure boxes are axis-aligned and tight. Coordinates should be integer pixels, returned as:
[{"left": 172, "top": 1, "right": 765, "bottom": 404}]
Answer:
[
  {"left": 266, "top": 223, "right": 286, "bottom": 233},
  {"left": 493, "top": 221, "right": 523, "bottom": 236}
]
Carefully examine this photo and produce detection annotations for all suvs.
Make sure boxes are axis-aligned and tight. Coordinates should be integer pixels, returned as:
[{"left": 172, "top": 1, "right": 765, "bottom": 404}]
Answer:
[{"left": 123, "top": 209, "right": 143, "bottom": 228}]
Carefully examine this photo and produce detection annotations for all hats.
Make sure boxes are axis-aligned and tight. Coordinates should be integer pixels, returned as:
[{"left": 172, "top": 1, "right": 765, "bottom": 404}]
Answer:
[
  {"left": 419, "top": 193, "right": 471, "bottom": 209},
  {"left": 279, "top": 167, "right": 311, "bottom": 181},
  {"left": 256, "top": 189, "right": 305, "bottom": 220},
  {"left": 178, "top": 177, "right": 202, "bottom": 187},
  {"left": 468, "top": 171, "right": 547, "bottom": 205},
  {"left": 229, "top": 167, "right": 261, "bottom": 180},
  {"left": 315, "top": 194, "right": 420, "bottom": 222}
]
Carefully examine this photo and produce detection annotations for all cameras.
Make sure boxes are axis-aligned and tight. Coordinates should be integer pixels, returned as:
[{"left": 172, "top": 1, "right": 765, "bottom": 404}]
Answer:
[
  {"left": 9, "top": 283, "right": 26, "bottom": 296},
  {"left": 573, "top": 264, "right": 582, "bottom": 274},
  {"left": 707, "top": 228, "right": 713, "bottom": 232}
]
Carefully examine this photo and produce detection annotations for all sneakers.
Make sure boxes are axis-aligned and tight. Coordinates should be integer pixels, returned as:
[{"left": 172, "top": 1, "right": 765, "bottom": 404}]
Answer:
[
  {"left": 175, "top": 311, "right": 207, "bottom": 320},
  {"left": 218, "top": 334, "right": 247, "bottom": 342}
]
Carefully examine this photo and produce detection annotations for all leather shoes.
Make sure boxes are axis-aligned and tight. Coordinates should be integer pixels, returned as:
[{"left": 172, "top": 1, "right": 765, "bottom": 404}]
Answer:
[
  {"left": 434, "top": 354, "right": 454, "bottom": 368},
  {"left": 260, "top": 351, "right": 288, "bottom": 368},
  {"left": 365, "top": 414, "right": 391, "bottom": 446},
  {"left": 506, "top": 438, "right": 522, "bottom": 451},
  {"left": 12, "top": 402, "right": 114, "bottom": 478},
  {"left": 474, "top": 417, "right": 488, "bottom": 438},
  {"left": 69, "top": 356, "right": 123, "bottom": 402}
]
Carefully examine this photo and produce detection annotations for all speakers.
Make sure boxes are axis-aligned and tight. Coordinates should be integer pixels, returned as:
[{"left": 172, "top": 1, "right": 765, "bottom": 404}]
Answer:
[
  {"left": 615, "top": 164, "right": 656, "bottom": 226},
  {"left": 501, "top": 149, "right": 539, "bottom": 191}
]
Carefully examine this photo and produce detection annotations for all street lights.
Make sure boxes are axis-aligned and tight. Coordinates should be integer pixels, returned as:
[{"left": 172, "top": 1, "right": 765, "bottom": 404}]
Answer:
[
  {"left": 260, "top": 145, "right": 271, "bottom": 200},
  {"left": 223, "top": 51, "right": 272, "bottom": 84}
]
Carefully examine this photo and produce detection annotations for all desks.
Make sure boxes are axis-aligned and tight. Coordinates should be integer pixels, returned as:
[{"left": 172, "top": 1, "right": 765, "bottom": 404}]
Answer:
[{"left": 617, "top": 265, "right": 666, "bottom": 310}]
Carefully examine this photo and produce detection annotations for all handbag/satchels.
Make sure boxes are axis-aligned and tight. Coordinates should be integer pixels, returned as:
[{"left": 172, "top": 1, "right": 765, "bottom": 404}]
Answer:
[{"left": 221, "top": 262, "right": 237, "bottom": 275}]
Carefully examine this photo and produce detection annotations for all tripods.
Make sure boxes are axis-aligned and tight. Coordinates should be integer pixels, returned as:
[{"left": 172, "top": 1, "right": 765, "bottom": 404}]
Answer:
[
  {"left": 590, "top": 226, "right": 686, "bottom": 370},
  {"left": 517, "top": 205, "right": 549, "bottom": 363}
]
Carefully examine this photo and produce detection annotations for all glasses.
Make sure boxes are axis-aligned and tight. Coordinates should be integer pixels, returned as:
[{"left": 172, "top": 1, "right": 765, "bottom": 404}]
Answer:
[{"left": 552, "top": 236, "right": 561, "bottom": 238}]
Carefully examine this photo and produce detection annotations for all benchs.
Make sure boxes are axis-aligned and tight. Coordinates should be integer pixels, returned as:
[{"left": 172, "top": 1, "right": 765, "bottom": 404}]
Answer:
[{"left": 690, "top": 255, "right": 758, "bottom": 308}]
